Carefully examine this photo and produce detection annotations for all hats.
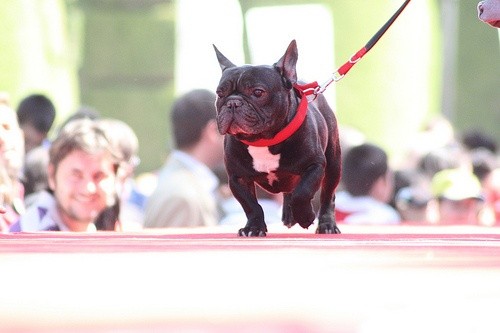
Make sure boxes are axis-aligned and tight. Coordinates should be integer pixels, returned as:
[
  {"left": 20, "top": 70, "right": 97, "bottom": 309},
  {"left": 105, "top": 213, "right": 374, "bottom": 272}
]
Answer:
[{"left": 430, "top": 167, "right": 486, "bottom": 202}]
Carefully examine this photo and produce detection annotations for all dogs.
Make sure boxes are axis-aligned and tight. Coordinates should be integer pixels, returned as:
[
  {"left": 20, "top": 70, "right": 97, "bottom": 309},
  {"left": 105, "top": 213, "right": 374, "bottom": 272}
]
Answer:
[{"left": 212, "top": 39, "right": 343, "bottom": 238}]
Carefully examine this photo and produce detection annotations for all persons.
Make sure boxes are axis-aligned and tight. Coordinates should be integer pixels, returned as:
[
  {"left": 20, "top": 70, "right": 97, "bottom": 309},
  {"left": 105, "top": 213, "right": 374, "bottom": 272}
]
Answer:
[
  {"left": 223, "top": 182, "right": 288, "bottom": 232},
  {"left": 144, "top": 88, "right": 225, "bottom": 228},
  {"left": 392, "top": 132, "right": 500, "bottom": 229},
  {"left": 10, "top": 109, "right": 152, "bottom": 233},
  {"left": 335, "top": 143, "right": 401, "bottom": 224},
  {"left": 18, "top": 95, "right": 56, "bottom": 152},
  {"left": 0, "top": 96, "right": 27, "bottom": 233}
]
